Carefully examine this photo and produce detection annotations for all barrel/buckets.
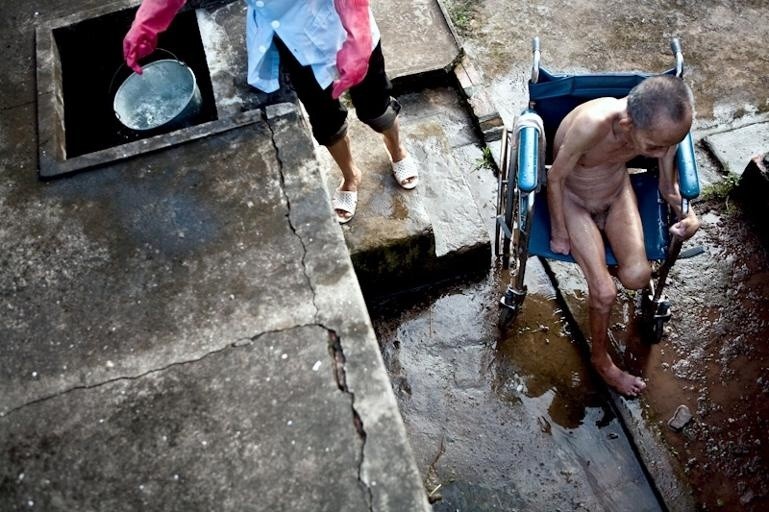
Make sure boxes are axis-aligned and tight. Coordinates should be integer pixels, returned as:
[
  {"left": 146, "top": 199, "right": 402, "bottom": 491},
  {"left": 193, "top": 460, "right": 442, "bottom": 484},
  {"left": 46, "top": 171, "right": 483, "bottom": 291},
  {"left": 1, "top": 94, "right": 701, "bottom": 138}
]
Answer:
[{"left": 106, "top": 47, "right": 203, "bottom": 136}]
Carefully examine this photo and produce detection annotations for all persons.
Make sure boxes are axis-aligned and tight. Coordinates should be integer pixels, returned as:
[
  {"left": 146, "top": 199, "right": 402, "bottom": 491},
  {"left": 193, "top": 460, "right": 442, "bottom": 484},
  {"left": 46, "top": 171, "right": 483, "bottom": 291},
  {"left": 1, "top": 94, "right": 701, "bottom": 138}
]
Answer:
[
  {"left": 123, "top": 0, "right": 418, "bottom": 223},
  {"left": 547, "top": 75, "right": 700, "bottom": 397}
]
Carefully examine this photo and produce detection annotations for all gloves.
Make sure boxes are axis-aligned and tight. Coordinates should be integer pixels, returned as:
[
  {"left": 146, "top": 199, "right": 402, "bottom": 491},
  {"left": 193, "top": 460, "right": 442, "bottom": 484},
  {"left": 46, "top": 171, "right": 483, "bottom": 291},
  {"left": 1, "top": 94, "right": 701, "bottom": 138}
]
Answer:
[
  {"left": 328, "top": 0, "right": 373, "bottom": 104},
  {"left": 121, "top": 0, "right": 187, "bottom": 76}
]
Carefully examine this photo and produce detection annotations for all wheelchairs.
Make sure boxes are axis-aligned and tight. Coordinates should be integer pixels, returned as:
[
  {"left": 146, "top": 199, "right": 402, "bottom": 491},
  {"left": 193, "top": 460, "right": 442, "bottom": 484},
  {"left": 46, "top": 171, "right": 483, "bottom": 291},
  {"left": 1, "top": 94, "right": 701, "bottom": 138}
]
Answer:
[{"left": 491, "top": 32, "right": 702, "bottom": 351}]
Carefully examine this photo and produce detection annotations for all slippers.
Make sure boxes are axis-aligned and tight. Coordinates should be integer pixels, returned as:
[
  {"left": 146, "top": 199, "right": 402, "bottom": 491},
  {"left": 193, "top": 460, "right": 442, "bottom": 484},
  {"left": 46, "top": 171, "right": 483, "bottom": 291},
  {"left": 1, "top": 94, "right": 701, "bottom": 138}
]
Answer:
[
  {"left": 331, "top": 165, "right": 363, "bottom": 225},
  {"left": 383, "top": 138, "right": 419, "bottom": 191}
]
